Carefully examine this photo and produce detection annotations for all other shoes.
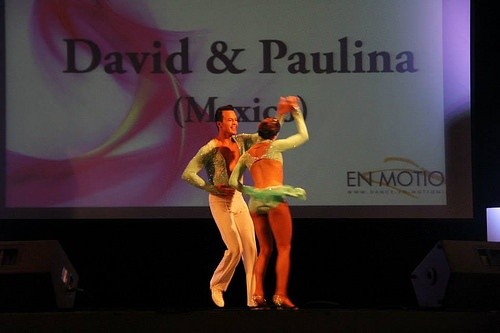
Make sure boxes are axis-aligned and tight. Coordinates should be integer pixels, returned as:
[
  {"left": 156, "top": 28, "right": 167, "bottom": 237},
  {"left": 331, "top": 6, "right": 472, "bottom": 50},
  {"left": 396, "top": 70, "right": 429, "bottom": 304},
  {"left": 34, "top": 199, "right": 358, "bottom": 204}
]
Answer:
[{"left": 212, "top": 288, "right": 225, "bottom": 308}]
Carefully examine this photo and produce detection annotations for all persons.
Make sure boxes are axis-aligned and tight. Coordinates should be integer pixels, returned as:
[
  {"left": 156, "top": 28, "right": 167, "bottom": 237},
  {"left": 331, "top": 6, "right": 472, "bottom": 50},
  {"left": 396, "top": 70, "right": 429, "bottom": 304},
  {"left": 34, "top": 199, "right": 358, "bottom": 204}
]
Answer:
[
  {"left": 181, "top": 97, "right": 292, "bottom": 307},
  {"left": 229, "top": 94, "right": 309, "bottom": 311}
]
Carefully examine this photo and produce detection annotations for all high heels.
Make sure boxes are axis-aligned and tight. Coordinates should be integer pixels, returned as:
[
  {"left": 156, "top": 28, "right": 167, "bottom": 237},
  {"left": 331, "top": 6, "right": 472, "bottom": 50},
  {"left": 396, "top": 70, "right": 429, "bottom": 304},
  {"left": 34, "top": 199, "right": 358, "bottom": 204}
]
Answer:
[
  {"left": 254, "top": 297, "right": 266, "bottom": 307},
  {"left": 273, "top": 295, "right": 298, "bottom": 312}
]
